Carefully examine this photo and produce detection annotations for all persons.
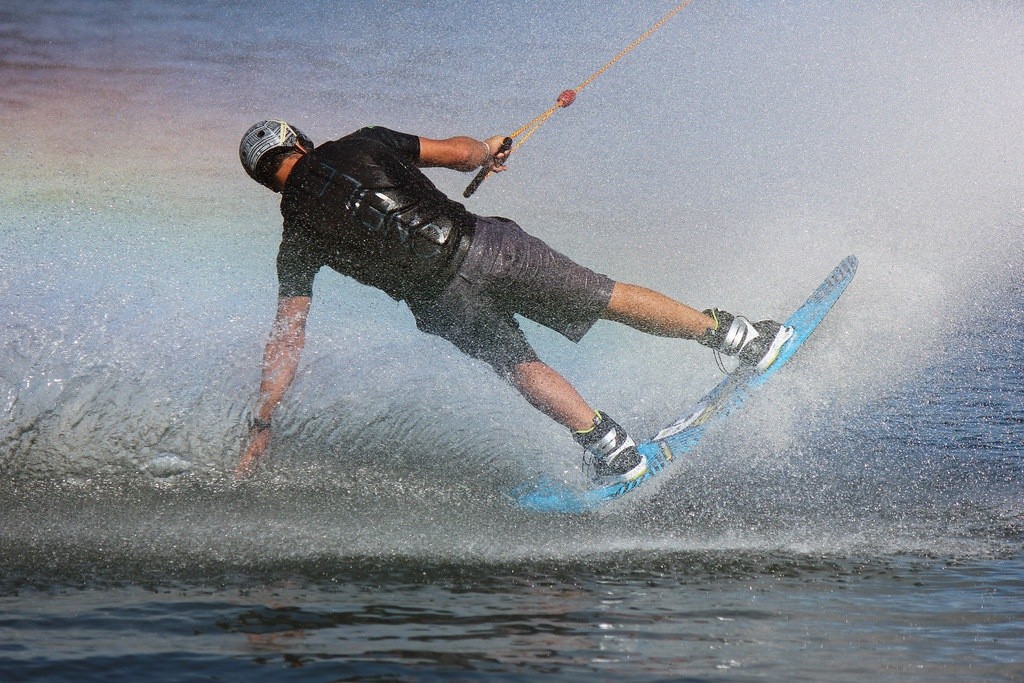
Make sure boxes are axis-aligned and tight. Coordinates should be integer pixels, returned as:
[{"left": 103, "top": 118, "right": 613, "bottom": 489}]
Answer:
[{"left": 237, "top": 120, "right": 795, "bottom": 487}]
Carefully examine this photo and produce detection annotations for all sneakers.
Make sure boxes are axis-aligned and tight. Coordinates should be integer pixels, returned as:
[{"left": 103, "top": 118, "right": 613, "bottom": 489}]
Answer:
[
  {"left": 698, "top": 306, "right": 786, "bottom": 372},
  {"left": 570, "top": 409, "right": 650, "bottom": 484}
]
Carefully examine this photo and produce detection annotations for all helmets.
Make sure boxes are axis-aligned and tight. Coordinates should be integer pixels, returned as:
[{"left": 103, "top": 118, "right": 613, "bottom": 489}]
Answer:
[{"left": 239, "top": 118, "right": 300, "bottom": 193}]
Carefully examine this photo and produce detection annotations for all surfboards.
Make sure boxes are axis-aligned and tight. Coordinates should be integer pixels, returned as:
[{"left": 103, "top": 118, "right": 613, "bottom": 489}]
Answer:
[{"left": 500, "top": 254, "right": 859, "bottom": 515}]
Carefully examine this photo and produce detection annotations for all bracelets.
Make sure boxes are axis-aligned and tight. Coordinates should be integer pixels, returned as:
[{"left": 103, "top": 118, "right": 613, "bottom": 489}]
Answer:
[
  {"left": 481, "top": 140, "right": 491, "bottom": 166},
  {"left": 246, "top": 417, "right": 271, "bottom": 434}
]
